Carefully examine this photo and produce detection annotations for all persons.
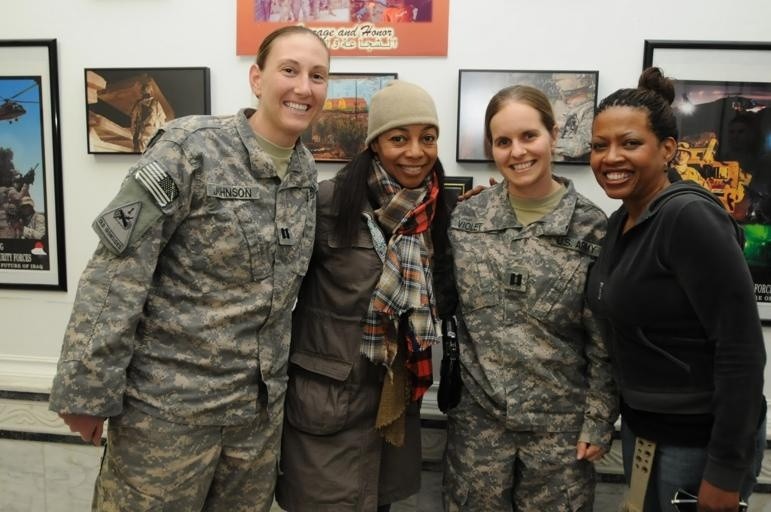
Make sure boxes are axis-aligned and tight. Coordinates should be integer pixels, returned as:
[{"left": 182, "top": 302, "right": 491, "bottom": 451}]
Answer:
[
  {"left": 723, "top": 115, "right": 771, "bottom": 184},
  {"left": 670, "top": 140, "right": 710, "bottom": 192},
  {"left": 130, "top": 84, "right": 166, "bottom": 154},
  {"left": 442, "top": 85, "right": 619, "bottom": 511},
  {"left": 7, "top": 171, "right": 31, "bottom": 238},
  {"left": 277, "top": 82, "right": 453, "bottom": 512},
  {"left": 48, "top": 27, "right": 331, "bottom": 511},
  {"left": 550, "top": 73, "right": 595, "bottom": 162},
  {"left": 458, "top": 68, "right": 768, "bottom": 512}
]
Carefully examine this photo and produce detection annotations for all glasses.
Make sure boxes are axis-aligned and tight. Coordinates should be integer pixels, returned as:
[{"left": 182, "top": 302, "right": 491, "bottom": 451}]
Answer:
[{"left": 671, "top": 490, "right": 748, "bottom": 512}]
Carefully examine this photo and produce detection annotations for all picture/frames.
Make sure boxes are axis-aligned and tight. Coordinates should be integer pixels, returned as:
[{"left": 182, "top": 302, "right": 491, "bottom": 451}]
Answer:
[
  {"left": 84, "top": 66, "right": 211, "bottom": 155},
  {"left": 456, "top": 69, "right": 599, "bottom": 165},
  {"left": 300, "top": 72, "right": 398, "bottom": 163},
  {"left": 0, "top": 38, "right": 67, "bottom": 291},
  {"left": 443, "top": 176, "right": 472, "bottom": 212},
  {"left": 642, "top": 39, "right": 771, "bottom": 326}
]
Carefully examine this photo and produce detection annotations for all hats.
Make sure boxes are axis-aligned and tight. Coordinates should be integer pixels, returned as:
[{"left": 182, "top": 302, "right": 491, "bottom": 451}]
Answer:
[{"left": 364, "top": 81, "right": 440, "bottom": 149}]
